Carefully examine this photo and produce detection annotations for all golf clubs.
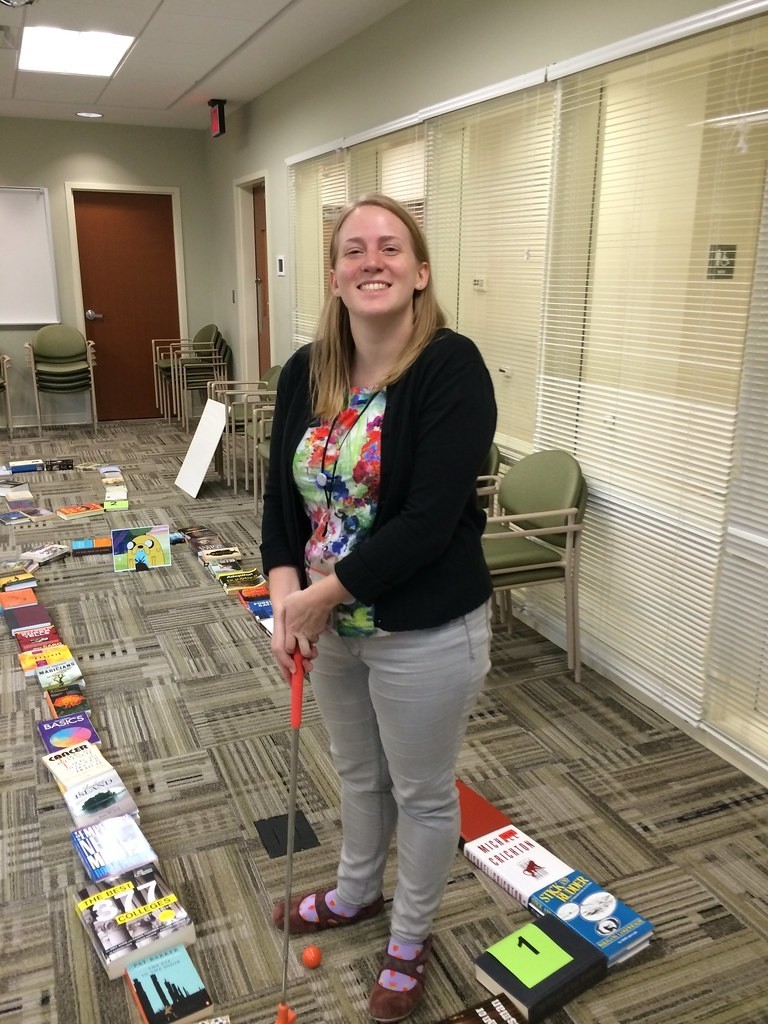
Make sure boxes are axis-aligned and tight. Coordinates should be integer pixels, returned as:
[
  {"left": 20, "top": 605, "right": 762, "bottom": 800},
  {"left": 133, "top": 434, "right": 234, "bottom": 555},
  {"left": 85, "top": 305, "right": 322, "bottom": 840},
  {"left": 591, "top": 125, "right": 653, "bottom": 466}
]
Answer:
[{"left": 272, "top": 644, "right": 305, "bottom": 1024}]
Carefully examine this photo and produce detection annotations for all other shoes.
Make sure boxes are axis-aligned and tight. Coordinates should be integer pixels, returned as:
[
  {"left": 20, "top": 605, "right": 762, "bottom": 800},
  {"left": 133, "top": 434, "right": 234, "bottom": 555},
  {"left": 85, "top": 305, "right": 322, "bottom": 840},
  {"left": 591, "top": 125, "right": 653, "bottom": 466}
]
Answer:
[
  {"left": 369, "top": 930, "right": 434, "bottom": 1021},
  {"left": 273, "top": 887, "right": 386, "bottom": 934}
]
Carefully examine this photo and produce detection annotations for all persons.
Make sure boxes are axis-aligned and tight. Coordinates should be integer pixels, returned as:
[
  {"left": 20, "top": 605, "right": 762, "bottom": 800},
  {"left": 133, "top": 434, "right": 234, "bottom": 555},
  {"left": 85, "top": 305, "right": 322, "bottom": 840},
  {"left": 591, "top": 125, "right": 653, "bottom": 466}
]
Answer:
[{"left": 257, "top": 193, "right": 499, "bottom": 1024}]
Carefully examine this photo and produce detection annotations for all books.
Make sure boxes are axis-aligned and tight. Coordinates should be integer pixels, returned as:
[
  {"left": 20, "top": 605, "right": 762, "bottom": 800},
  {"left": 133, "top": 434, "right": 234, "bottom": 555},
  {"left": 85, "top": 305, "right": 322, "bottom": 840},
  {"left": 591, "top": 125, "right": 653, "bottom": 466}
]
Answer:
[{"left": 0, "top": 459, "right": 653, "bottom": 1024}]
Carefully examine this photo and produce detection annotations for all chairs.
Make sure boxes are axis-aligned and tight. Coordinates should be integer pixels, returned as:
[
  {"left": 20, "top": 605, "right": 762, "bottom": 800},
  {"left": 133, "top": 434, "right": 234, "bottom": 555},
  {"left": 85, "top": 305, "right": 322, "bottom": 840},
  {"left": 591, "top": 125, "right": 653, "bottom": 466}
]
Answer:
[
  {"left": 0, "top": 324, "right": 98, "bottom": 438},
  {"left": 152, "top": 324, "right": 588, "bottom": 684}
]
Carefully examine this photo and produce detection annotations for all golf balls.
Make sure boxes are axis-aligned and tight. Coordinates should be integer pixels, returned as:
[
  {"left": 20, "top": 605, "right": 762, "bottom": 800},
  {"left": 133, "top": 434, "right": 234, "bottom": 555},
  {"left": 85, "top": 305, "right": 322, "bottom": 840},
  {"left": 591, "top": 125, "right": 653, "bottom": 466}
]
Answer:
[{"left": 302, "top": 945, "right": 322, "bottom": 969}]
